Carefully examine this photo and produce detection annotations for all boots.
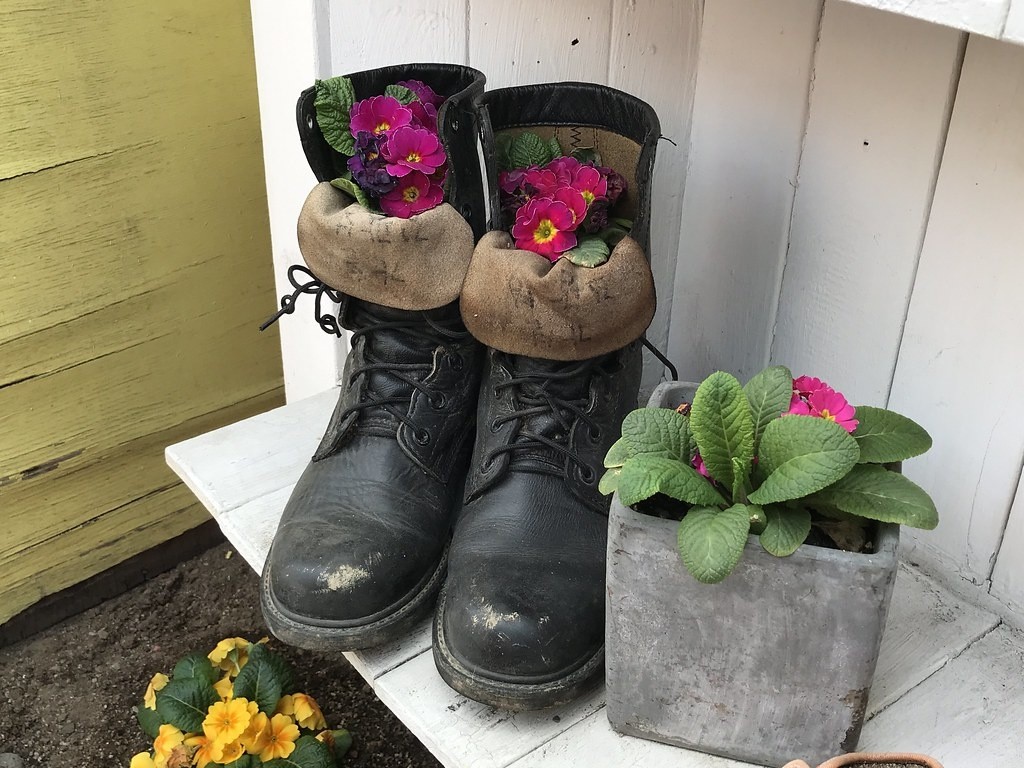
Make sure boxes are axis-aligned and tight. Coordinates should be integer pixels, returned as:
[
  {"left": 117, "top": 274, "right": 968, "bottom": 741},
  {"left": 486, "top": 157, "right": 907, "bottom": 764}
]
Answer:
[
  {"left": 258, "top": 61, "right": 489, "bottom": 652},
  {"left": 433, "top": 83, "right": 681, "bottom": 713}
]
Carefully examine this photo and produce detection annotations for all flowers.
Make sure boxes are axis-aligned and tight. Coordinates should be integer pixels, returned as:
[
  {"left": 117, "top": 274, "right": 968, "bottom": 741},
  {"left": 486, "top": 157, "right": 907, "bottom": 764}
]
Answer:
[
  {"left": 313, "top": 73, "right": 456, "bottom": 218},
  {"left": 492, "top": 130, "right": 635, "bottom": 269},
  {"left": 595, "top": 364, "right": 939, "bottom": 584},
  {"left": 128, "top": 635, "right": 353, "bottom": 768}
]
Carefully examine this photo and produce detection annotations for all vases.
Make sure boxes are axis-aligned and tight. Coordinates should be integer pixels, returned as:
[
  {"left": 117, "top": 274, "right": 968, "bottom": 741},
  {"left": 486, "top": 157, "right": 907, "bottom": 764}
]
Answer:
[{"left": 604, "top": 378, "right": 902, "bottom": 768}]
